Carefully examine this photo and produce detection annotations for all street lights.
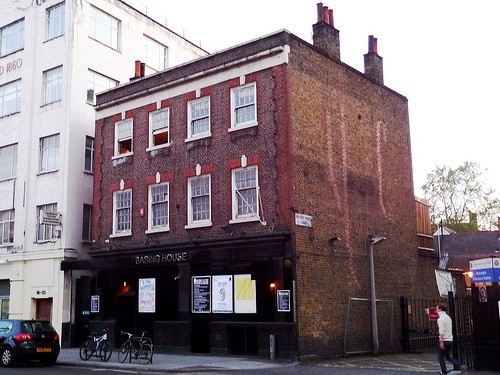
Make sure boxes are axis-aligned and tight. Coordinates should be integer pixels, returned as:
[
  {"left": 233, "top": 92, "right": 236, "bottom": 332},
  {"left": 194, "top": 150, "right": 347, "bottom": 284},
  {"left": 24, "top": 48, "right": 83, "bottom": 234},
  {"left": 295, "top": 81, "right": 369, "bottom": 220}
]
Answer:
[{"left": 369, "top": 236, "right": 386, "bottom": 355}]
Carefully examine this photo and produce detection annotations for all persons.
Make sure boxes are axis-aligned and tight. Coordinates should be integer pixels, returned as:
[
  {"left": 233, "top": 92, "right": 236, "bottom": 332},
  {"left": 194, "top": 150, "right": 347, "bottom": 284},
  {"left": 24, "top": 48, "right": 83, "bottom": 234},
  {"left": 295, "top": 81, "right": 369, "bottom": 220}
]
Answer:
[{"left": 435, "top": 304, "right": 462, "bottom": 375}]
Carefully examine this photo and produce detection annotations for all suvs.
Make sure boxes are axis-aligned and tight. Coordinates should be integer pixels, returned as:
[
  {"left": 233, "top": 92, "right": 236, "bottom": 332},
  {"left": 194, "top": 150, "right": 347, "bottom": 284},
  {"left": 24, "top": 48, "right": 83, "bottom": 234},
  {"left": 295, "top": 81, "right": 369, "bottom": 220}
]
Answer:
[{"left": 0, "top": 318, "right": 61, "bottom": 367}]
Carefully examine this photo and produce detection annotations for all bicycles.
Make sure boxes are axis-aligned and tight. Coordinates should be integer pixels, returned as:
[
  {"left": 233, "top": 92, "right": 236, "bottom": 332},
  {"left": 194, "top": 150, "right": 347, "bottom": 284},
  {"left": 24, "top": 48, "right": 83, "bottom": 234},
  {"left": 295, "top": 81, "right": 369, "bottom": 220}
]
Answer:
[
  {"left": 117, "top": 331, "right": 153, "bottom": 365},
  {"left": 79, "top": 328, "right": 115, "bottom": 362}
]
